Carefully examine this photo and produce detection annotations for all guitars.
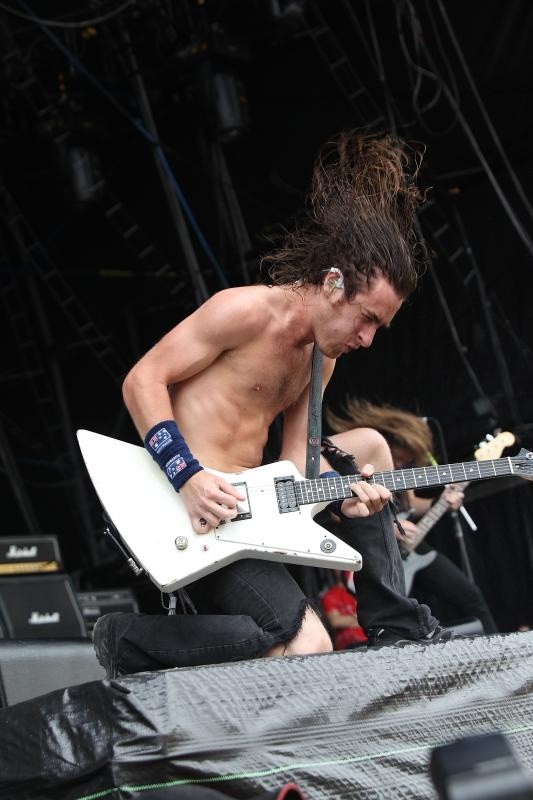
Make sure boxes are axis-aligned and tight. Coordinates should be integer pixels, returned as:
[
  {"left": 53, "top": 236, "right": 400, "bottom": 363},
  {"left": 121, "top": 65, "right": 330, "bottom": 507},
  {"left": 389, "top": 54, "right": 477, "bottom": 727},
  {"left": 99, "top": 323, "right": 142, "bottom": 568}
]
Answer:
[
  {"left": 75, "top": 427, "right": 533, "bottom": 596},
  {"left": 347, "top": 431, "right": 516, "bottom": 596}
]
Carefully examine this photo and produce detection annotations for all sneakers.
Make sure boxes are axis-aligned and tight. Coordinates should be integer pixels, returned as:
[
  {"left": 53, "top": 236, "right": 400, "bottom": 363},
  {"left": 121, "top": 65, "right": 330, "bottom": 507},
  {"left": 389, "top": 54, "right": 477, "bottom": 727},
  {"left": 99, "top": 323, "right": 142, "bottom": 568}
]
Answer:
[
  {"left": 92, "top": 612, "right": 121, "bottom": 679},
  {"left": 368, "top": 625, "right": 482, "bottom": 650}
]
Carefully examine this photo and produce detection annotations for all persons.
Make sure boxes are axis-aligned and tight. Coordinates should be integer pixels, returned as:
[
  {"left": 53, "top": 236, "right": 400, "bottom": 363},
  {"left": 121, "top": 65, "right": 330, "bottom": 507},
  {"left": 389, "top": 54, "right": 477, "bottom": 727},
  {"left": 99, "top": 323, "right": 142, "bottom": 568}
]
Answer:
[
  {"left": 92, "top": 135, "right": 447, "bottom": 681},
  {"left": 319, "top": 399, "right": 498, "bottom": 650}
]
